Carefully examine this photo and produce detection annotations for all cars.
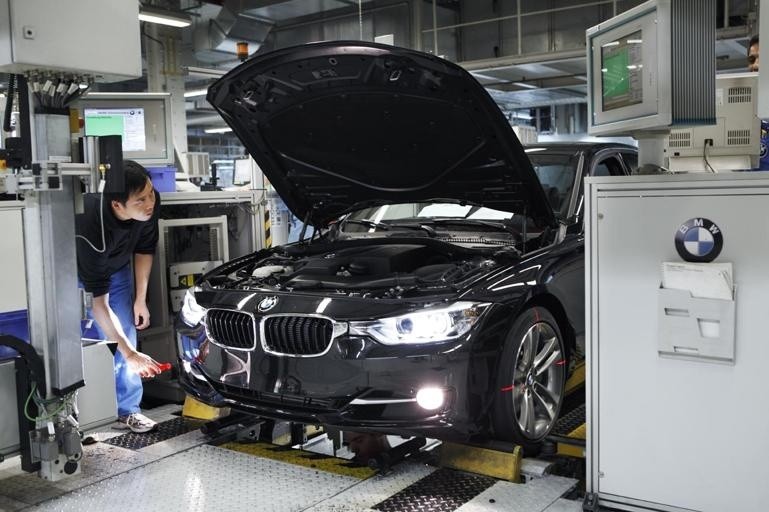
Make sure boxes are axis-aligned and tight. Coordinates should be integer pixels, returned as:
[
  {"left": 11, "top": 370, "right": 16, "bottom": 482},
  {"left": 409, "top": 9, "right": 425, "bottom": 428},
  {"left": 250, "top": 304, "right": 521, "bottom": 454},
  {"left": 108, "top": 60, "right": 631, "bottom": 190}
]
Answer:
[{"left": 173, "top": 39, "right": 638, "bottom": 444}]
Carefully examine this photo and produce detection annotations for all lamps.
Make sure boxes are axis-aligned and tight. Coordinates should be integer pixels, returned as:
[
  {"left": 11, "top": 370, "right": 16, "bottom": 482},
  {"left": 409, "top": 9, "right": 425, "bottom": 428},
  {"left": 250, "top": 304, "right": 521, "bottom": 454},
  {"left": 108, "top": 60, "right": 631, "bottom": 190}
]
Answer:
[
  {"left": 183, "top": 85, "right": 210, "bottom": 98},
  {"left": 204, "top": 126, "right": 233, "bottom": 134},
  {"left": 138, "top": 7, "right": 193, "bottom": 28}
]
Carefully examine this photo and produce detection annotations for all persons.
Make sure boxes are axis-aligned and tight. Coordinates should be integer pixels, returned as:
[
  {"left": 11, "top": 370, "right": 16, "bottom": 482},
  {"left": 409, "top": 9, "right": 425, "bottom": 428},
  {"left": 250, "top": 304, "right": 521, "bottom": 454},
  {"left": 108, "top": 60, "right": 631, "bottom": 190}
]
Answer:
[
  {"left": 74, "top": 159, "right": 162, "bottom": 435},
  {"left": 744, "top": 36, "right": 768, "bottom": 171}
]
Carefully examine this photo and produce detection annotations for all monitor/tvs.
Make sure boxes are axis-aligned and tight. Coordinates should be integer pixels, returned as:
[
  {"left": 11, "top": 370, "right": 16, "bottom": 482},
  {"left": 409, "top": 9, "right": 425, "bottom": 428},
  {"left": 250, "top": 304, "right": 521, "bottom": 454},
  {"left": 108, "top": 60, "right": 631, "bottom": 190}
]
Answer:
[
  {"left": 84, "top": 92, "right": 175, "bottom": 166},
  {"left": 591, "top": 8, "right": 658, "bottom": 125}
]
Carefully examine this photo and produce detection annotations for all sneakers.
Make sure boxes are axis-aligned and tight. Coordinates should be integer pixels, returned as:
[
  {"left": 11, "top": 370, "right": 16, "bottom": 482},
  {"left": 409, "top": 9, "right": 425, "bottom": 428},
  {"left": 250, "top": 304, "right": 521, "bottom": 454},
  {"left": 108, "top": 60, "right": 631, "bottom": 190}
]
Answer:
[
  {"left": 81, "top": 431, "right": 99, "bottom": 445},
  {"left": 111, "top": 412, "right": 159, "bottom": 434}
]
